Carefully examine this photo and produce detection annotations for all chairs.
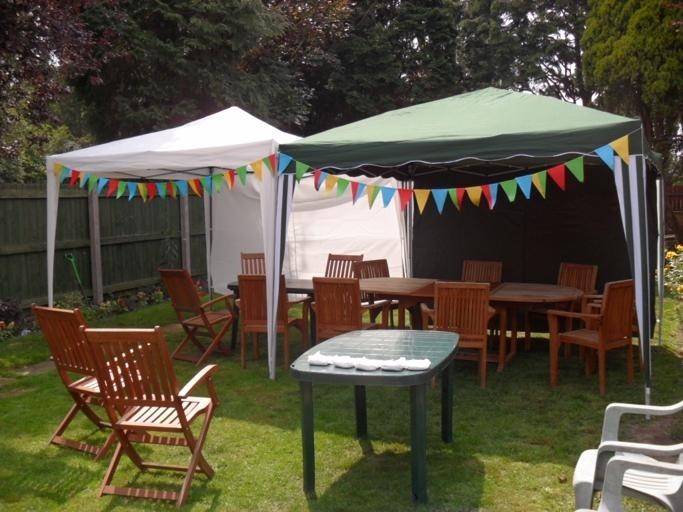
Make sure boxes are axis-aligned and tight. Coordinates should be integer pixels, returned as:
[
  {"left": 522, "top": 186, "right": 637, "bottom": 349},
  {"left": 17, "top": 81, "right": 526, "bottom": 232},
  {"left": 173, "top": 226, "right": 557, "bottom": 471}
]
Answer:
[
  {"left": 158, "top": 269, "right": 236, "bottom": 366},
  {"left": 354, "top": 260, "right": 414, "bottom": 330},
  {"left": 526, "top": 261, "right": 598, "bottom": 360},
  {"left": 571, "top": 398, "right": 683, "bottom": 512},
  {"left": 420, "top": 282, "right": 496, "bottom": 389},
  {"left": 548, "top": 278, "right": 635, "bottom": 395},
  {"left": 461, "top": 260, "right": 503, "bottom": 353},
  {"left": 310, "top": 275, "right": 378, "bottom": 346},
  {"left": 238, "top": 275, "right": 307, "bottom": 370},
  {"left": 584, "top": 294, "right": 644, "bottom": 372},
  {"left": 574, "top": 455, "right": 683, "bottom": 512},
  {"left": 240, "top": 252, "right": 266, "bottom": 275},
  {"left": 79, "top": 326, "right": 223, "bottom": 508},
  {"left": 32, "top": 303, "right": 150, "bottom": 461},
  {"left": 303, "top": 252, "right": 364, "bottom": 319}
]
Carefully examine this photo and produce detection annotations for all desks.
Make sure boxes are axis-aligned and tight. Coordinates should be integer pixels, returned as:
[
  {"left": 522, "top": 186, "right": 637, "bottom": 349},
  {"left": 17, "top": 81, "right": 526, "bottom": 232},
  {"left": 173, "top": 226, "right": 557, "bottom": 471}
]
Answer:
[
  {"left": 226, "top": 279, "right": 314, "bottom": 351},
  {"left": 288, "top": 331, "right": 462, "bottom": 500},
  {"left": 358, "top": 277, "right": 584, "bottom": 386}
]
